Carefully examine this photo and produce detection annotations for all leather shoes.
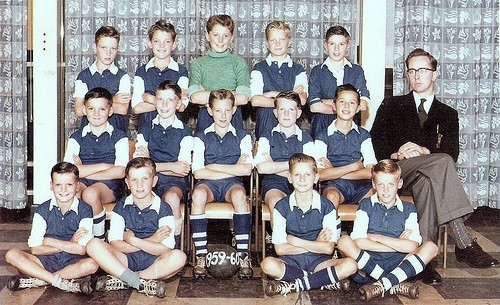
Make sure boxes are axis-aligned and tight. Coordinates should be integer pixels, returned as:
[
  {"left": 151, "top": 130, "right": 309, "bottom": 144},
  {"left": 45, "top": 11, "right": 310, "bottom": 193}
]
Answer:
[
  {"left": 454, "top": 241, "right": 500, "bottom": 269},
  {"left": 423, "top": 263, "right": 443, "bottom": 286}
]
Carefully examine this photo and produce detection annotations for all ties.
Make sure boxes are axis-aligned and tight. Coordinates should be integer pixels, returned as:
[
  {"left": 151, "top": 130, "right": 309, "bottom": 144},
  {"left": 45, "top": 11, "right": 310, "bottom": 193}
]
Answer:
[{"left": 417, "top": 99, "right": 428, "bottom": 130}]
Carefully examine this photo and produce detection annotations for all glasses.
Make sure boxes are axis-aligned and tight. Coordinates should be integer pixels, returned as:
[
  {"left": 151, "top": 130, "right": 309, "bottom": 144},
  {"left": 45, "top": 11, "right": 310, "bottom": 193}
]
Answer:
[{"left": 407, "top": 68, "right": 435, "bottom": 76}]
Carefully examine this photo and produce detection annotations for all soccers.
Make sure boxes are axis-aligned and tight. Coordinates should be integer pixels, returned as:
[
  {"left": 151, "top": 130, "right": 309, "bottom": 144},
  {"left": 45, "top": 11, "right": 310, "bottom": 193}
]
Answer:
[{"left": 205, "top": 243, "right": 239, "bottom": 279}]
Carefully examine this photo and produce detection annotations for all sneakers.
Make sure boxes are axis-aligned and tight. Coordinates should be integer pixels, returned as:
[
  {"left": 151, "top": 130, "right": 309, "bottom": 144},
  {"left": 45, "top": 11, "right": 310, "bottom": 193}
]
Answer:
[
  {"left": 322, "top": 278, "right": 350, "bottom": 292},
  {"left": 194, "top": 256, "right": 207, "bottom": 279},
  {"left": 237, "top": 256, "right": 253, "bottom": 280},
  {"left": 265, "top": 278, "right": 296, "bottom": 297},
  {"left": 137, "top": 278, "right": 166, "bottom": 298},
  {"left": 67, "top": 279, "right": 93, "bottom": 295},
  {"left": 359, "top": 280, "right": 386, "bottom": 301},
  {"left": 392, "top": 279, "right": 418, "bottom": 298},
  {"left": 8, "top": 275, "right": 34, "bottom": 291},
  {"left": 96, "top": 275, "right": 128, "bottom": 292}
]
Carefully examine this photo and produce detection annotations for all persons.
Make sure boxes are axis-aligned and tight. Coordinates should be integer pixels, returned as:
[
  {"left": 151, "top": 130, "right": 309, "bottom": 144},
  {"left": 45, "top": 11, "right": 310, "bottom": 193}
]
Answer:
[
  {"left": 5, "top": 162, "right": 102, "bottom": 295},
  {"left": 75, "top": 25, "right": 131, "bottom": 131},
  {"left": 61, "top": 87, "right": 131, "bottom": 245},
  {"left": 187, "top": 14, "right": 254, "bottom": 246},
  {"left": 259, "top": 153, "right": 358, "bottom": 297},
  {"left": 308, "top": 26, "right": 371, "bottom": 139},
  {"left": 250, "top": 22, "right": 310, "bottom": 254},
  {"left": 253, "top": 91, "right": 317, "bottom": 233},
  {"left": 368, "top": 47, "right": 499, "bottom": 287},
  {"left": 131, "top": 19, "right": 192, "bottom": 135},
  {"left": 128, "top": 80, "right": 193, "bottom": 253},
  {"left": 312, "top": 84, "right": 376, "bottom": 240},
  {"left": 84, "top": 157, "right": 187, "bottom": 298},
  {"left": 188, "top": 88, "right": 255, "bottom": 281},
  {"left": 338, "top": 160, "right": 440, "bottom": 302}
]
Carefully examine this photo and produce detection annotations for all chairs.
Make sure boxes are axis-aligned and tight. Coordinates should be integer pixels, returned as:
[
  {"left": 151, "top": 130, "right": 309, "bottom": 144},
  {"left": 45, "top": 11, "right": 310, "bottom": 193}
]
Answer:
[{"left": 100, "top": 140, "right": 449, "bottom": 271}]
